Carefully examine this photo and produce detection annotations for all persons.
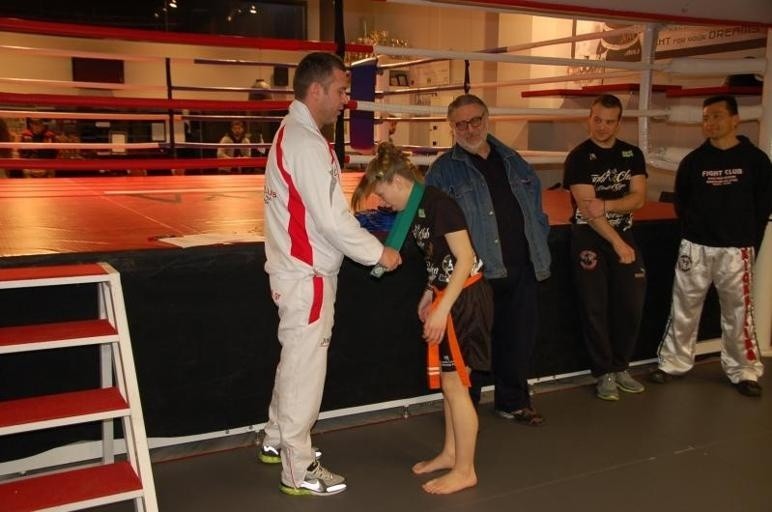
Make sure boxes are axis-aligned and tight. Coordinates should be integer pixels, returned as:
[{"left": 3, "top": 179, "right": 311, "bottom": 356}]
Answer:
[
  {"left": 217, "top": 120, "right": 252, "bottom": 173},
  {"left": 426, "top": 95, "right": 551, "bottom": 426},
  {"left": 17, "top": 116, "right": 59, "bottom": 179},
  {"left": 650, "top": 94, "right": 772, "bottom": 396},
  {"left": 351, "top": 141, "right": 493, "bottom": 494},
  {"left": 0, "top": 117, "right": 23, "bottom": 177},
  {"left": 563, "top": 94, "right": 648, "bottom": 400},
  {"left": 258, "top": 53, "right": 401, "bottom": 498}
]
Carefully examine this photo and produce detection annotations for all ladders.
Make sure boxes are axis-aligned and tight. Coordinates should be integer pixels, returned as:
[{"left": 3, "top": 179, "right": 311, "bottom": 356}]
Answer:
[{"left": 0, "top": 261, "right": 161, "bottom": 512}]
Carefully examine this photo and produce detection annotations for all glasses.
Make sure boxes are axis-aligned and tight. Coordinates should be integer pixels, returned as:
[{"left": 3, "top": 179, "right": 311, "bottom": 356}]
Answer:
[{"left": 453, "top": 111, "right": 484, "bottom": 130}]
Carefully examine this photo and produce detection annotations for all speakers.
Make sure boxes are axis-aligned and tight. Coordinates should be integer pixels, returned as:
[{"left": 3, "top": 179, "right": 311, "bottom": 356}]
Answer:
[{"left": 274, "top": 65, "right": 289, "bottom": 86}]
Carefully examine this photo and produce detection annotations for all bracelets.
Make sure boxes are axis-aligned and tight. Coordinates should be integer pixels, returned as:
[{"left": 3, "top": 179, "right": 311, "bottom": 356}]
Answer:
[{"left": 603, "top": 199, "right": 609, "bottom": 219}]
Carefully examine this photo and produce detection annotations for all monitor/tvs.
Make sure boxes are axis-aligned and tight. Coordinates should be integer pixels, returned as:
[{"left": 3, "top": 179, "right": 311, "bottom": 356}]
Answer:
[{"left": 72, "top": 57, "right": 125, "bottom": 84}]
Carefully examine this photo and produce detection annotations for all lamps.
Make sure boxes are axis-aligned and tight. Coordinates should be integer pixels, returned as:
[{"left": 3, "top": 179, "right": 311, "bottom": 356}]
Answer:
[{"left": 248, "top": 0, "right": 274, "bottom": 102}]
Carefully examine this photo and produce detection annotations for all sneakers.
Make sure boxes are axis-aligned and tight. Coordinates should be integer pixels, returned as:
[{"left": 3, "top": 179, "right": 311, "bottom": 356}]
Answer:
[
  {"left": 281, "top": 461, "right": 348, "bottom": 495},
  {"left": 737, "top": 378, "right": 761, "bottom": 398},
  {"left": 498, "top": 407, "right": 540, "bottom": 427},
  {"left": 650, "top": 368, "right": 676, "bottom": 382},
  {"left": 593, "top": 372, "right": 620, "bottom": 402},
  {"left": 257, "top": 443, "right": 322, "bottom": 466},
  {"left": 616, "top": 372, "right": 645, "bottom": 394}
]
